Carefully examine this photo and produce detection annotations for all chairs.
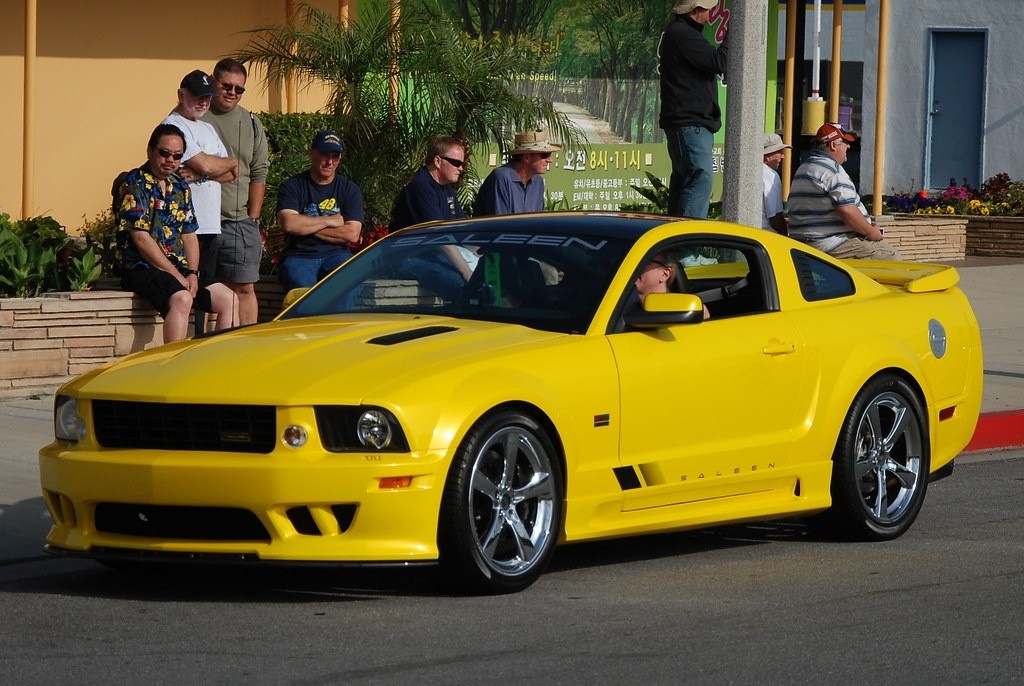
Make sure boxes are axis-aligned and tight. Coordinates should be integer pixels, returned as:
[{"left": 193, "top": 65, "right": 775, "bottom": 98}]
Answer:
[{"left": 672, "top": 261, "right": 690, "bottom": 296}]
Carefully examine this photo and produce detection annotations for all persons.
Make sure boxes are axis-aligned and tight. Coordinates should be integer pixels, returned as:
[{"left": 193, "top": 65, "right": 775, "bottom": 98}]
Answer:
[
  {"left": 114, "top": 124, "right": 240, "bottom": 344},
  {"left": 275, "top": 129, "right": 366, "bottom": 311},
  {"left": 634, "top": 249, "right": 709, "bottom": 331},
  {"left": 761, "top": 133, "right": 793, "bottom": 236},
  {"left": 475, "top": 130, "right": 582, "bottom": 303},
  {"left": 786, "top": 122, "right": 903, "bottom": 262},
  {"left": 658, "top": 0, "right": 731, "bottom": 219},
  {"left": 198, "top": 58, "right": 271, "bottom": 332},
  {"left": 162, "top": 69, "right": 241, "bottom": 335},
  {"left": 389, "top": 136, "right": 492, "bottom": 305}
]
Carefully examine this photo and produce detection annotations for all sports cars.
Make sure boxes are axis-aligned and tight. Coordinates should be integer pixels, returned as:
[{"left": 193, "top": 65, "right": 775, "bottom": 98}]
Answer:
[{"left": 38, "top": 210, "right": 983, "bottom": 594}]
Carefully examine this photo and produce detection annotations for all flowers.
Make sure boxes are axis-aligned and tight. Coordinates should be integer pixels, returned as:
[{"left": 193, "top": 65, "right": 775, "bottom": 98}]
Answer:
[{"left": 887, "top": 171, "right": 1024, "bottom": 216}]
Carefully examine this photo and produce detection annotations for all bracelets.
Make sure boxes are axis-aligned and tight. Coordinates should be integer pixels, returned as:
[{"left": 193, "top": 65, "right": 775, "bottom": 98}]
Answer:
[
  {"left": 251, "top": 218, "right": 260, "bottom": 224},
  {"left": 195, "top": 174, "right": 210, "bottom": 183}
]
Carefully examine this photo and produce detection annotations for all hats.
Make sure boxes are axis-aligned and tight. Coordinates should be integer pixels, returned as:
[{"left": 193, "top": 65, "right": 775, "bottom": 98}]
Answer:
[
  {"left": 180, "top": 69, "right": 219, "bottom": 97},
  {"left": 816, "top": 123, "right": 855, "bottom": 144},
  {"left": 312, "top": 130, "right": 343, "bottom": 153},
  {"left": 503, "top": 130, "right": 562, "bottom": 155},
  {"left": 671, "top": 0, "right": 718, "bottom": 15},
  {"left": 763, "top": 133, "right": 793, "bottom": 154}
]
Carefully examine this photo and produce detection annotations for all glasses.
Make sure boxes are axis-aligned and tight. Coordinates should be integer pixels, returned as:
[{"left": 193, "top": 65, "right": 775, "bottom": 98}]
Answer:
[
  {"left": 156, "top": 145, "right": 183, "bottom": 160},
  {"left": 440, "top": 157, "right": 466, "bottom": 169},
  {"left": 214, "top": 76, "right": 245, "bottom": 94},
  {"left": 541, "top": 153, "right": 551, "bottom": 159}
]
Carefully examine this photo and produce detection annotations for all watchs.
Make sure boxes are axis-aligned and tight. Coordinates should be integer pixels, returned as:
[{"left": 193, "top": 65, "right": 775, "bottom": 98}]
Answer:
[{"left": 184, "top": 269, "right": 201, "bottom": 279}]
